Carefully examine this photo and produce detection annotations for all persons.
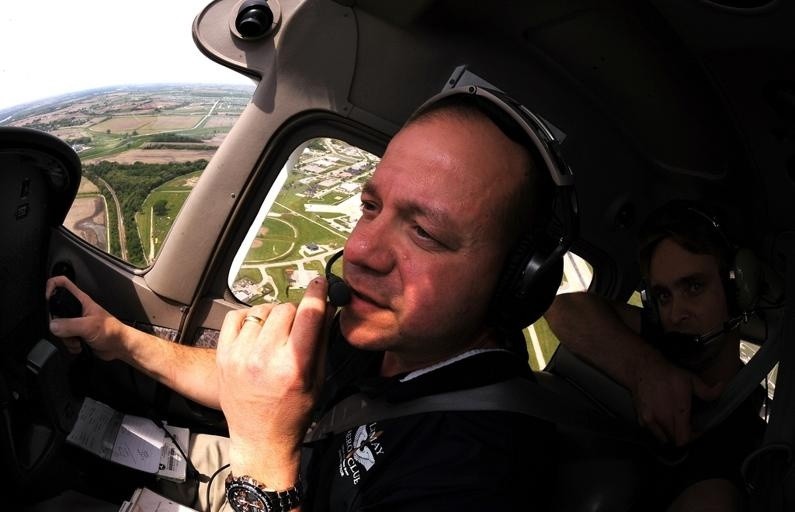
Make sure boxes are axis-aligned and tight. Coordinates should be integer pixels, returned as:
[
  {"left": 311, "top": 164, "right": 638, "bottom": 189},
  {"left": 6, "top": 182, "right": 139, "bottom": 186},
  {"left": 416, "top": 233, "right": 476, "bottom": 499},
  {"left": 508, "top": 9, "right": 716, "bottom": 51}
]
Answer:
[
  {"left": 542, "top": 206, "right": 767, "bottom": 512},
  {"left": 40, "top": 91, "right": 554, "bottom": 512}
]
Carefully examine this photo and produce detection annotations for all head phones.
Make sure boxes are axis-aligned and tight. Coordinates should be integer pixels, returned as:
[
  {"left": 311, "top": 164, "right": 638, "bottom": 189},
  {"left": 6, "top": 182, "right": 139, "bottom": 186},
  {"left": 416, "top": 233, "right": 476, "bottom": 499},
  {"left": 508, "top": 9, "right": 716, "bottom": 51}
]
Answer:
[
  {"left": 399, "top": 82, "right": 580, "bottom": 331},
  {"left": 638, "top": 209, "right": 762, "bottom": 329}
]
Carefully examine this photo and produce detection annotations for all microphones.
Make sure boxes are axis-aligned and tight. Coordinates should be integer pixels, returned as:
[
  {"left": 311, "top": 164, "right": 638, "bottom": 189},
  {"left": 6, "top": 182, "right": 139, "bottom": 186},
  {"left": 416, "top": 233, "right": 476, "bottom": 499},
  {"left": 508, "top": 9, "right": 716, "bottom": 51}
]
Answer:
[
  {"left": 686, "top": 310, "right": 755, "bottom": 354},
  {"left": 325, "top": 250, "right": 352, "bottom": 308}
]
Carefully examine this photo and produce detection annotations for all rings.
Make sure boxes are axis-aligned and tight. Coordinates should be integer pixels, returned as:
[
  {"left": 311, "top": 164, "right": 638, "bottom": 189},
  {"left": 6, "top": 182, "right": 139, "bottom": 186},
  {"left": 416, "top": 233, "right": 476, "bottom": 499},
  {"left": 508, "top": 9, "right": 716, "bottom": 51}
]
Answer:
[{"left": 241, "top": 316, "right": 264, "bottom": 327}]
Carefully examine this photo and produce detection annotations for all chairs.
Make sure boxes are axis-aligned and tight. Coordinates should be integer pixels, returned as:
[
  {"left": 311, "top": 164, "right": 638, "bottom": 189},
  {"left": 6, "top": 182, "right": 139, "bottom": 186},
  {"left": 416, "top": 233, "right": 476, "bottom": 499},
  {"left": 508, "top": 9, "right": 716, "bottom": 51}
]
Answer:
[{"left": 533, "top": 344, "right": 644, "bottom": 511}]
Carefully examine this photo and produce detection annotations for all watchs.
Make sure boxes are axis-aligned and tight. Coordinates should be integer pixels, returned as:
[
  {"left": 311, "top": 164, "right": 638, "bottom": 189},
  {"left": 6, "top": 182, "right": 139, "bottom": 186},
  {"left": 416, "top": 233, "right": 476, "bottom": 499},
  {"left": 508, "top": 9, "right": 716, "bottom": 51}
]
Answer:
[{"left": 224, "top": 475, "right": 302, "bottom": 512}]
